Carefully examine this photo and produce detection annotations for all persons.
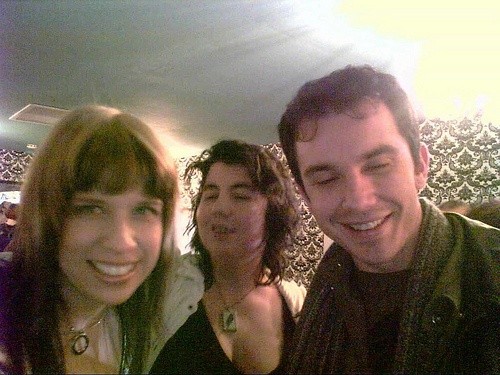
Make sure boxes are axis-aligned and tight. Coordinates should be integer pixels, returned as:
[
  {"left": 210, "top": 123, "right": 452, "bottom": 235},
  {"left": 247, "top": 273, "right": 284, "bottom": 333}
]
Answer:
[
  {"left": 436, "top": 200, "right": 470, "bottom": 218},
  {"left": 0, "top": 105, "right": 238, "bottom": 374},
  {"left": 463, "top": 199, "right": 500, "bottom": 228},
  {"left": 179, "top": 137, "right": 311, "bottom": 375},
  {"left": 275, "top": 63, "right": 500, "bottom": 375},
  {"left": 0, "top": 200, "right": 14, "bottom": 225},
  {"left": 0, "top": 204, "right": 22, "bottom": 251}
]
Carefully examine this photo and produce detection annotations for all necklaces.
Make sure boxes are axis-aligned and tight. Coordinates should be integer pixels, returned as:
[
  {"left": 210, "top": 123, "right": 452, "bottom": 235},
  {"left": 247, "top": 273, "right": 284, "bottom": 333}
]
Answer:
[
  {"left": 60, "top": 300, "right": 111, "bottom": 356},
  {"left": 209, "top": 272, "right": 265, "bottom": 332}
]
